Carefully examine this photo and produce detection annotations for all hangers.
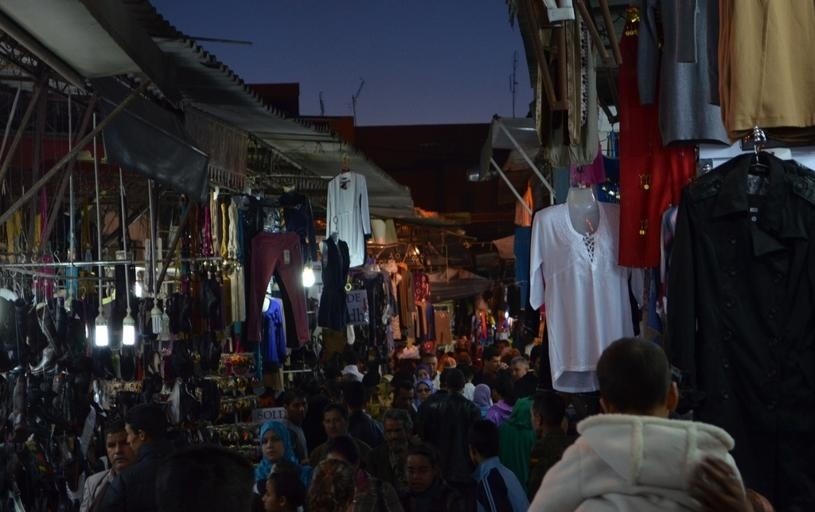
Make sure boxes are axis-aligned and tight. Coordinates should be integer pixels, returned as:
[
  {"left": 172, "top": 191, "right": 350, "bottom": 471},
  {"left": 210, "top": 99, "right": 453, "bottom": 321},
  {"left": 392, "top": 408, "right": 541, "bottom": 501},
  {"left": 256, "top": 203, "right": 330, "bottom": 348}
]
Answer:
[
  {"left": 340, "top": 169, "right": 350, "bottom": 183},
  {"left": 746, "top": 129, "right": 769, "bottom": 176},
  {"left": 366, "top": 240, "right": 453, "bottom": 345},
  {"left": 0, "top": 257, "right": 236, "bottom": 511}
]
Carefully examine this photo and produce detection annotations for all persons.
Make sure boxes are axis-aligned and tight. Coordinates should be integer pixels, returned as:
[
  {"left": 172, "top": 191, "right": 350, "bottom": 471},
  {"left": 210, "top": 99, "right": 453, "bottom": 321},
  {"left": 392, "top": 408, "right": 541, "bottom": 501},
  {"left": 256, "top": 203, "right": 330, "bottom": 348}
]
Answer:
[
  {"left": 86, "top": 403, "right": 214, "bottom": 512},
  {"left": 308, "top": 408, "right": 389, "bottom": 486},
  {"left": 81, "top": 422, "right": 136, "bottom": 512},
  {"left": 305, "top": 377, "right": 331, "bottom": 409},
  {"left": 419, "top": 425, "right": 473, "bottom": 500},
  {"left": 527, "top": 396, "right": 580, "bottom": 502},
  {"left": 484, "top": 384, "right": 517, "bottom": 429},
  {"left": 509, "top": 360, "right": 541, "bottom": 403},
  {"left": 319, "top": 437, "right": 404, "bottom": 512},
  {"left": 473, "top": 384, "right": 495, "bottom": 421},
  {"left": 341, "top": 382, "right": 385, "bottom": 450},
  {"left": 321, "top": 367, "right": 346, "bottom": 403},
  {"left": 339, "top": 353, "right": 365, "bottom": 382},
  {"left": 468, "top": 420, "right": 530, "bottom": 512},
  {"left": 475, "top": 349, "right": 505, "bottom": 389},
  {"left": 526, "top": 338, "right": 747, "bottom": 512},
  {"left": 422, "top": 357, "right": 445, "bottom": 390},
  {"left": 252, "top": 424, "right": 312, "bottom": 511},
  {"left": 263, "top": 473, "right": 305, "bottom": 512},
  {"left": 393, "top": 384, "right": 428, "bottom": 444},
  {"left": 458, "top": 353, "right": 480, "bottom": 403},
  {"left": 396, "top": 450, "right": 468, "bottom": 512},
  {"left": 277, "top": 392, "right": 309, "bottom": 469},
  {"left": 436, "top": 337, "right": 521, "bottom": 368},
  {"left": 374, "top": 411, "right": 430, "bottom": 486},
  {"left": 306, "top": 460, "right": 355, "bottom": 511},
  {"left": 414, "top": 370, "right": 478, "bottom": 457}
]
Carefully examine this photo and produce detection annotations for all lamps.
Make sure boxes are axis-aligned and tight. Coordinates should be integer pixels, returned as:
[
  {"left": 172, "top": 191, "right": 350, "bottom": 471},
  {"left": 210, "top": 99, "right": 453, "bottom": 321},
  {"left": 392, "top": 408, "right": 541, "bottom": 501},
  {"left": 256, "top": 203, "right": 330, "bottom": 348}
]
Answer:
[
  {"left": 93, "top": 112, "right": 110, "bottom": 347},
  {"left": 118, "top": 168, "right": 136, "bottom": 345},
  {"left": 64, "top": 95, "right": 75, "bottom": 312},
  {"left": 135, "top": 270, "right": 146, "bottom": 298},
  {"left": 161, "top": 314, "right": 169, "bottom": 335},
  {"left": 148, "top": 178, "right": 162, "bottom": 334}
]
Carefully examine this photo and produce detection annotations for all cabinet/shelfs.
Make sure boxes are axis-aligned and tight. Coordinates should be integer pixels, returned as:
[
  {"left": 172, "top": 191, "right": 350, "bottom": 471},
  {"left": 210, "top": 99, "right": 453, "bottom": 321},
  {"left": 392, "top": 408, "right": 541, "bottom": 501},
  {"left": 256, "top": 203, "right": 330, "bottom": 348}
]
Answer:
[{"left": 213, "top": 353, "right": 265, "bottom": 469}]
